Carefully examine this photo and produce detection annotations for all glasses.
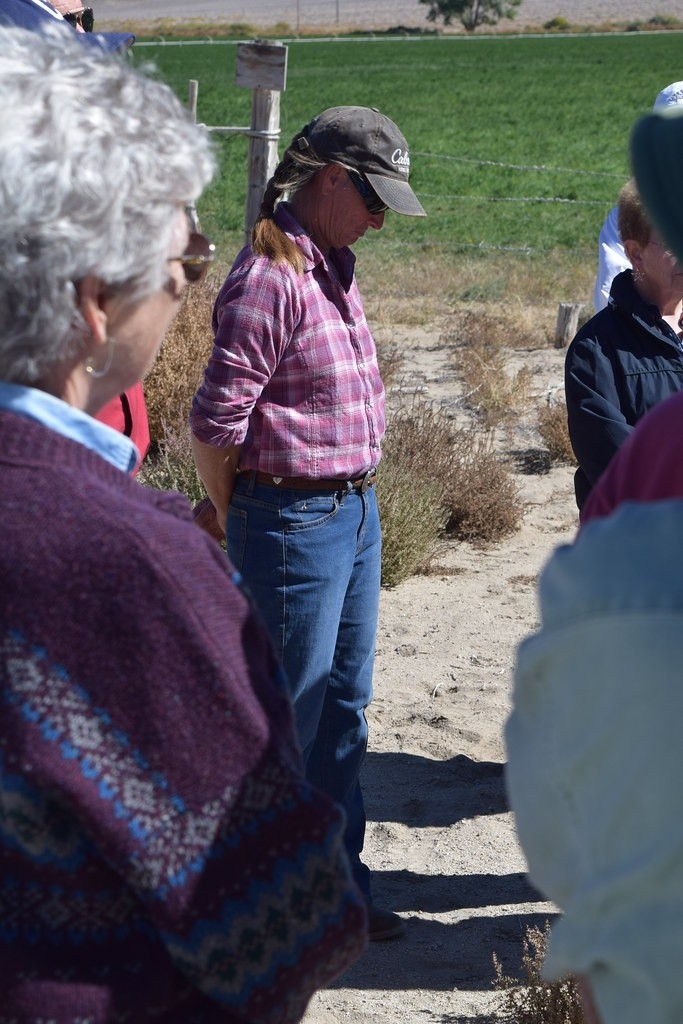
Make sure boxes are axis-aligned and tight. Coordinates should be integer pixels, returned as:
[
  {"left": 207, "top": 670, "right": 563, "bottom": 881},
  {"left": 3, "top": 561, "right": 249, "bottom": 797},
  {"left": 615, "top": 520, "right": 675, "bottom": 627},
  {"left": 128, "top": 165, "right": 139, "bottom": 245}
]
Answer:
[
  {"left": 62, "top": 6, "right": 95, "bottom": 34},
  {"left": 168, "top": 228, "right": 217, "bottom": 284},
  {"left": 347, "top": 169, "right": 390, "bottom": 216}
]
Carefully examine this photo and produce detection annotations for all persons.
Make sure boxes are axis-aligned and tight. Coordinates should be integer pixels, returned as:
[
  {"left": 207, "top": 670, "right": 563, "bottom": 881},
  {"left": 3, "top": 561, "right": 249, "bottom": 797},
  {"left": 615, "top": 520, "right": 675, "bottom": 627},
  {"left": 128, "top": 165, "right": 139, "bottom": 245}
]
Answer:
[
  {"left": 502, "top": 80, "right": 683, "bottom": 1024},
  {"left": 1, "top": 0, "right": 430, "bottom": 1021}
]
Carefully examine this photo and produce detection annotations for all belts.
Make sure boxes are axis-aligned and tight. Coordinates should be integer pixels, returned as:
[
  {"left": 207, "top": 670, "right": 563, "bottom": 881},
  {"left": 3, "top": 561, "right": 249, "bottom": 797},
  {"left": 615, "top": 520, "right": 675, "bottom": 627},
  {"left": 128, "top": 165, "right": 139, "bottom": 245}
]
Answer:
[{"left": 244, "top": 470, "right": 381, "bottom": 493}]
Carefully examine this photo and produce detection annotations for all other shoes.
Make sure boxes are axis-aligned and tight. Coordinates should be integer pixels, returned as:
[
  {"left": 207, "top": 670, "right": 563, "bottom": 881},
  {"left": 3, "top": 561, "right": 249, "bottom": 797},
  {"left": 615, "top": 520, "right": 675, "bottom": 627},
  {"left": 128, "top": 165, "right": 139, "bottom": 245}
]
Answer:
[{"left": 364, "top": 904, "right": 409, "bottom": 943}]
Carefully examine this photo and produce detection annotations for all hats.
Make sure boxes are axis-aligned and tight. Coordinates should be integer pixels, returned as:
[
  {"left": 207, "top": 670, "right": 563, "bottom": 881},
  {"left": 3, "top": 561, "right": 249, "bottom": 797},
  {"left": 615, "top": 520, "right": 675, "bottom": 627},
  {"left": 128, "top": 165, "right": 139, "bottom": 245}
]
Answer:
[
  {"left": 0, "top": 0, "right": 137, "bottom": 55},
  {"left": 653, "top": 79, "right": 683, "bottom": 115},
  {"left": 303, "top": 106, "right": 427, "bottom": 216}
]
